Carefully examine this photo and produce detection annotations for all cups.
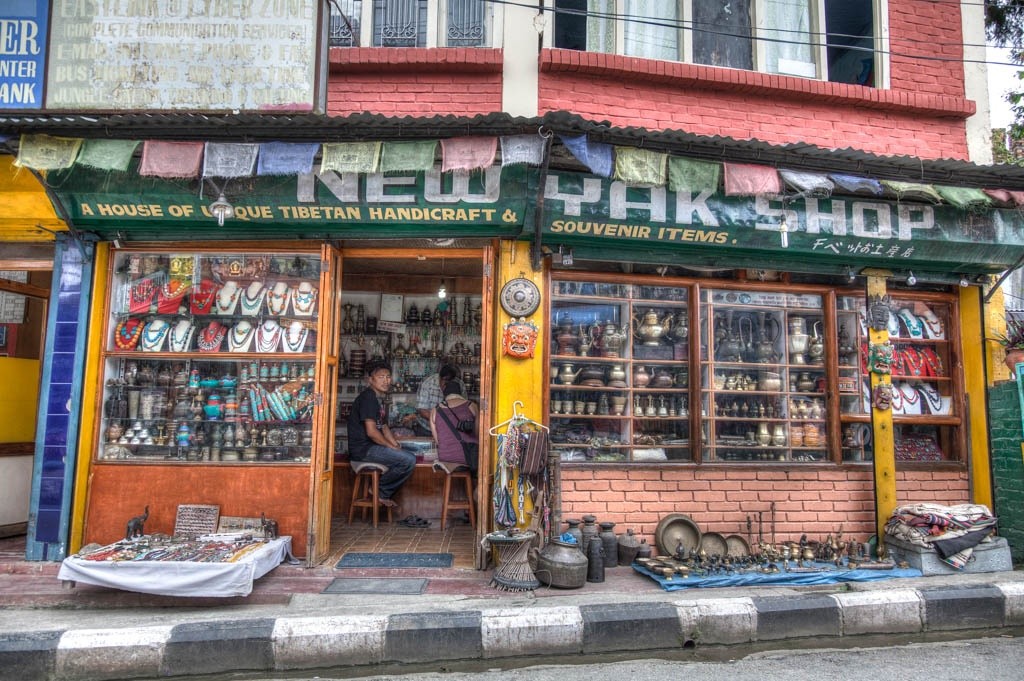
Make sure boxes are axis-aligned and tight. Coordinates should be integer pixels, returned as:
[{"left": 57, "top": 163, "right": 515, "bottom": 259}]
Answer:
[
  {"left": 635, "top": 555, "right": 690, "bottom": 581},
  {"left": 550, "top": 393, "right": 688, "bottom": 417}
]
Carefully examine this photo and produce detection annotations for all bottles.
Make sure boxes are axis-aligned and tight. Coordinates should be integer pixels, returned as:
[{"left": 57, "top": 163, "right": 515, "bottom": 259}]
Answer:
[
  {"left": 531, "top": 535, "right": 589, "bottom": 588},
  {"left": 99, "top": 358, "right": 317, "bottom": 463},
  {"left": 338, "top": 293, "right": 480, "bottom": 394},
  {"left": 586, "top": 537, "right": 605, "bottom": 583},
  {"left": 566, "top": 519, "right": 583, "bottom": 550},
  {"left": 714, "top": 396, "right": 825, "bottom": 460},
  {"left": 618, "top": 527, "right": 652, "bottom": 567},
  {"left": 600, "top": 522, "right": 618, "bottom": 568},
  {"left": 582, "top": 515, "right": 599, "bottom": 557}
]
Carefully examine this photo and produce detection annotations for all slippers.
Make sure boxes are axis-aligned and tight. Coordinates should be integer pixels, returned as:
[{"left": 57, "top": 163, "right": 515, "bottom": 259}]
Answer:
[
  {"left": 396, "top": 515, "right": 417, "bottom": 526},
  {"left": 407, "top": 517, "right": 432, "bottom": 528}
]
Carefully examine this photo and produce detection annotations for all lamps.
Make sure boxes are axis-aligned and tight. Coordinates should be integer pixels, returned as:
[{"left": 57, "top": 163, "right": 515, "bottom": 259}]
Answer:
[{"left": 209, "top": 177, "right": 234, "bottom": 227}]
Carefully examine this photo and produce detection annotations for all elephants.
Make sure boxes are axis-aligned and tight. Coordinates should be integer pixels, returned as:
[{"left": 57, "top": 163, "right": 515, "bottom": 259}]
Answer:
[{"left": 127, "top": 505, "right": 149, "bottom": 540}]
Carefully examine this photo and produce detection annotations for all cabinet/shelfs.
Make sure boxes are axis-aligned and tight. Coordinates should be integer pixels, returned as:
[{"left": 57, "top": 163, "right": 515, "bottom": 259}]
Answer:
[
  {"left": 337, "top": 319, "right": 481, "bottom": 438},
  {"left": 90, "top": 238, "right": 321, "bottom": 464},
  {"left": 860, "top": 293, "right": 965, "bottom": 465},
  {"left": 550, "top": 276, "right": 863, "bottom": 470}
]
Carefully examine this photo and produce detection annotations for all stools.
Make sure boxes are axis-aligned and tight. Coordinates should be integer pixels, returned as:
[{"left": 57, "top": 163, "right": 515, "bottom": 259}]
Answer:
[
  {"left": 435, "top": 461, "right": 476, "bottom": 532},
  {"left": 348, "top": 461, "right": 393, "bottom": 525}
]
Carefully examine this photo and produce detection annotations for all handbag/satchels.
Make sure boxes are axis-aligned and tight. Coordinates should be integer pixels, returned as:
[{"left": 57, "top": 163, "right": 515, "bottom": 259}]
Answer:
[{"left": 462, "top": 442, "right": 478, "bottom": 471}]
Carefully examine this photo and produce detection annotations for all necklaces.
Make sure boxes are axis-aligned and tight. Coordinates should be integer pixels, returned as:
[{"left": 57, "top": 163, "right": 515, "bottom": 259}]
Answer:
[
  {"left": 131, "top": 282, "right": 318, "bottom": 316},
  {"left": 114, "top": 318, "right": 306, "bottom": 354},
  {"left": 893, "top": 347, "right": 946, "bottom": 374},
  {"left": 888, "top": 308, "right": 947, "bottom": 339},
  {"left": 74, "top": 540, "right": 264, "bottom": 563},
  {"left": 891, "top": 382, "right": 942, "bottom": 411}
]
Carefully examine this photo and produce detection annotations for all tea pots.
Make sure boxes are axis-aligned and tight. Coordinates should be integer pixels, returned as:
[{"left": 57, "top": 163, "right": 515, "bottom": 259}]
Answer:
[{"left": 548, "top": 301, "right": 859, "bottom": 394}]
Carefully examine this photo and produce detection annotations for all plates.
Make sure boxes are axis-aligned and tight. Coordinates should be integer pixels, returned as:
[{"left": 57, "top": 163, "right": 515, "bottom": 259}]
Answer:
[
  {"left": 725, "top": 535, "right": 750, "bottom": 559},
  {"left": 697, "top": 532, "right": 728, "bottom": 560}
]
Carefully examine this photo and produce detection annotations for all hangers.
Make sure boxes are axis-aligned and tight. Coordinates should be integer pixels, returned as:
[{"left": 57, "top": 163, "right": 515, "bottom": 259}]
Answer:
[{"left": 488, "top": 400, "right": 549, "bottom": 437}]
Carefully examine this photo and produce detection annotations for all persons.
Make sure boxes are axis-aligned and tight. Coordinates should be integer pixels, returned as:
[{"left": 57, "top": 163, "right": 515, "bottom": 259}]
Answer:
[
  {"left": 347, "top": 358, "right": 416, "bottom": 507},
  {"left": 416, "top": 366, "right": 480, "bottom": 518}
]
